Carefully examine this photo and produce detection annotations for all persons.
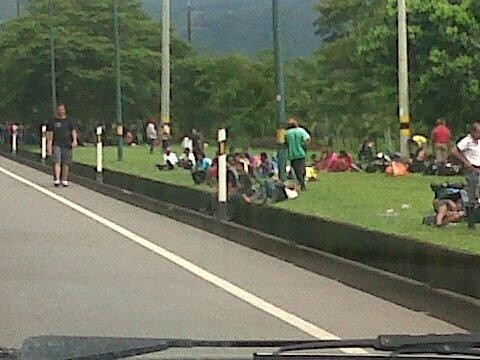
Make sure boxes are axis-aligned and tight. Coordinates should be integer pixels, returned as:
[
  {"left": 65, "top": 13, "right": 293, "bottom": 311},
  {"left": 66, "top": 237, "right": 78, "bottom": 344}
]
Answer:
[
  {"left": 45, "top": 103, "right": 78, "bottom": 187},
  {"left": 92, "top": 118, "right": 480, "bottom": 231}
]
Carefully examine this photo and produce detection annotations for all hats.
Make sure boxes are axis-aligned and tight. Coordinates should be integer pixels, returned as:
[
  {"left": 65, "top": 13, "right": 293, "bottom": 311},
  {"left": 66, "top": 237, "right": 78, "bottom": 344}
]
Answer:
[{"left": 286, "top": 118, "right": 297, "bottom": 127}]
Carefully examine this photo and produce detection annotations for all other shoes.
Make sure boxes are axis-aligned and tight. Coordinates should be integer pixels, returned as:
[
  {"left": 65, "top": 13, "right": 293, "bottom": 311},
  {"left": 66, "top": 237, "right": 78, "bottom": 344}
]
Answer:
[
  {"left": 54, "top": 181, "right": 60, "bottom": 185},
  {"left": 61, "top": 181, "right": 67, "bottom": 186}
]
